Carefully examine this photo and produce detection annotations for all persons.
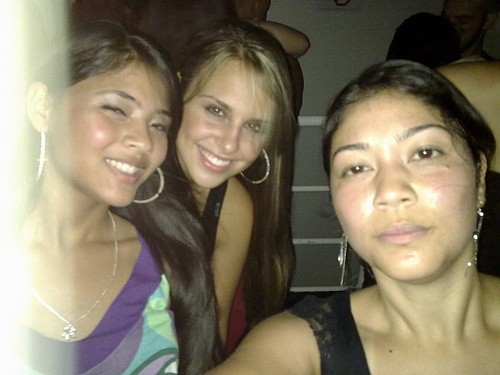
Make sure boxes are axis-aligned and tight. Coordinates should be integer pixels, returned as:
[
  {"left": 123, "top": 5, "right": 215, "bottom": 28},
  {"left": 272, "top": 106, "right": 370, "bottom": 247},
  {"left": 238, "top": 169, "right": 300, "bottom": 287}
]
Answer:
[
  {"left": 201, "top": 58, "right": 499, "bottom": 375},
  {"left": 0, "top": 22, "right": 182, "bottom": 375},
  {"left": 118, "top": 22, "right": 297, "bottom": 372},
  {"left": 384, "top": 13, "right": 499, "bottom": 276},
  {"left": 438, "top": 1, "right": 499, "bottom": 62},
  {"left": 191, "top": 0, "right": 311, "bottom": 60}
]
